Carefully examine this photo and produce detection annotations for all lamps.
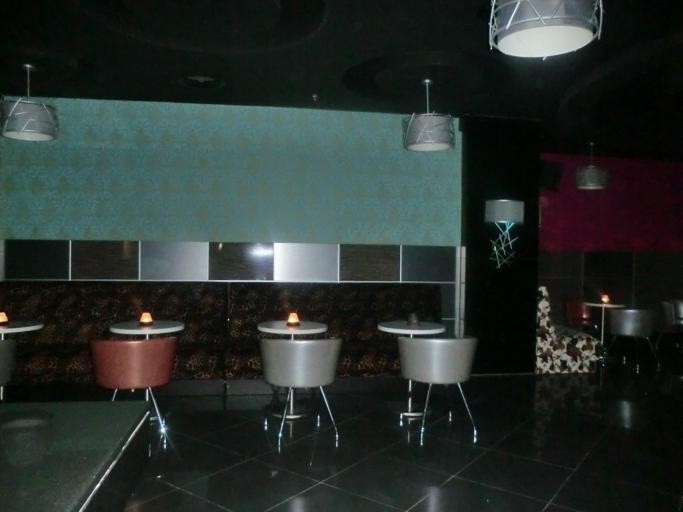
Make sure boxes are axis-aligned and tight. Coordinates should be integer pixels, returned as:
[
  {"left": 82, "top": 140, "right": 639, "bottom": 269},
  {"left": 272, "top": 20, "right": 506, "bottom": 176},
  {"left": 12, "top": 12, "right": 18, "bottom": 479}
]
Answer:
[
  {"left": 3, "top": 64, "right": 57, "bottom": 142},
  {"left": 401, "top": 80, "right": 454, "bottom": 151},
  {"left": 487, "top": 1, "right": 603, "bottom": 59},
  {"left": 483, "top": 199, "right": 523, "bottom": 267},
  {"left": 576, "top": 141, "right": 606, "bottom": 189}
]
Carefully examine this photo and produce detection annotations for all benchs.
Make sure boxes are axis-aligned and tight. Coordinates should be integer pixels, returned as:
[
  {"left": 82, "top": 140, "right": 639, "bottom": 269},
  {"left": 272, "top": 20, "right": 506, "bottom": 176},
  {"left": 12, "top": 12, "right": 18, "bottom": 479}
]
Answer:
[
  {"left": 535, "top": 373, "right": 598, "bottom": 451},
  {"left": 537, "top": 286, "right": 603, "bottom": 374}
]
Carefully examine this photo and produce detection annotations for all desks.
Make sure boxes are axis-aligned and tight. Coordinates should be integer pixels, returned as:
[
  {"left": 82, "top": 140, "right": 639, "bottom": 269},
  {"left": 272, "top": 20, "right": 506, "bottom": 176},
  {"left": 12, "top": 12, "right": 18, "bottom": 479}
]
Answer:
[
  {"left": 255, "top": 320, "right": 328, "bottom": 420},
  {"left": 375, "top": 321, "right": 445, "bottom": 416},
  {"left": 0, "top": 319, "right": 44, "bottom": 341},
  {"left": 107, "top": 319, "right": 184, "bottom": 421}
]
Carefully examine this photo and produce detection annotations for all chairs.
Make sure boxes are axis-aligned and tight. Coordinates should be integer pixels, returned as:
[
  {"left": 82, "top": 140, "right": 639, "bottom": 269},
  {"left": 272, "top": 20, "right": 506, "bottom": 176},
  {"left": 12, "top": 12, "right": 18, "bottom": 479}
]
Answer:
[
  {"left": 563, "top": 296, "right": 683, "bottom": 370},
  {"left": 0, "top": 339, "right": 15, "bottom": 399},
  {"left": 88, "top": 335, "right": 176, "bottom": 428},
  {"left": 260, "top": 339, "right": 338, "bottom": 436},
  {"left": 395, "top": 336, "right": 481, "bottom": 430}
]
